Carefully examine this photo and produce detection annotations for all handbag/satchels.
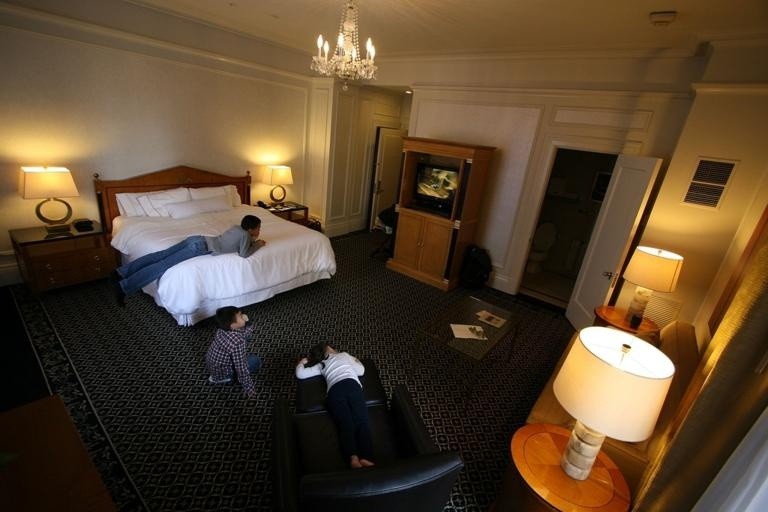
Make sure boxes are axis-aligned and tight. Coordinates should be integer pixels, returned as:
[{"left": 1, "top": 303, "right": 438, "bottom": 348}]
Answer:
[{"left": 463, "top": 243, "right": 492, "bottom": 287}]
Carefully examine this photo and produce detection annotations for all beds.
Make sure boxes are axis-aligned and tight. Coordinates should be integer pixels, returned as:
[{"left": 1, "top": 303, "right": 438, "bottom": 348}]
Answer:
[{"left": 93, "top": 164, "right": 335, "bottom": 327}]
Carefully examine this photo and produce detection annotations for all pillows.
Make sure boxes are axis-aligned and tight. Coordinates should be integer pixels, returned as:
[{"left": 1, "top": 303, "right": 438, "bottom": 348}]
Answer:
[{"left": 115, "top": 182, "right": 242, "bottom": 218}]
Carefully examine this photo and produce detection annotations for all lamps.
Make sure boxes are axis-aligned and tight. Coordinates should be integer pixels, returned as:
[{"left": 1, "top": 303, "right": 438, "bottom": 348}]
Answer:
[
  {"left": 17, "top": 166, "right": 80, "bottom": 224},
  {"left": 622, "top": 245, "right": 683, "bottom": 321},
  {"left": 266, "top": 165, "right": 294, "bottom": 201},
  {"left": 551, "top": 324, "right": 678, "bottom": 482},
  {"left": 311, "top": 0, "right": 379, "bottom": 91}
]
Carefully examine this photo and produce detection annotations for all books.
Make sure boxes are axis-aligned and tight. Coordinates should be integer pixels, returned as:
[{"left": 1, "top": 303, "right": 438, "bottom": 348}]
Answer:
[
  {"left": 476, "top": 309, "right": 489, "bottom": 317},
  {"left": 477, "top": 313, "right": 507, "bottom": 328},
  {"left": 449, "top": 323, "right": 488, "bottom": 341}
]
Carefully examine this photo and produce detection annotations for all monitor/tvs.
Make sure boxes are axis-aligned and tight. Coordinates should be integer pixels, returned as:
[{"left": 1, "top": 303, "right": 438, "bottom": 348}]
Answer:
[{"left": 405, "top": 163, "right": 459, "bottom": 219}]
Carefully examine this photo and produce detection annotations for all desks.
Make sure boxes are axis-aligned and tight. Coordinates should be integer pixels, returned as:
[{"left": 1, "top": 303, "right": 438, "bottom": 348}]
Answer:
[
  {"left": 0, "top": 392, "right": 115, "bottom": 512},
  {"left": 511, "top": 424, "right": 633, "bottom": 512},
  {"left": 591, "top": 305, "right": 661, "bottom": 339}
]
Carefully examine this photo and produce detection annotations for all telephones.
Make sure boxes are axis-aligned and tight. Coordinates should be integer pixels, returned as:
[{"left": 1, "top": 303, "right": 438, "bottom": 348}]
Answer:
[{"left": 258, "top": 201, "right": 271, "bottom": 209}]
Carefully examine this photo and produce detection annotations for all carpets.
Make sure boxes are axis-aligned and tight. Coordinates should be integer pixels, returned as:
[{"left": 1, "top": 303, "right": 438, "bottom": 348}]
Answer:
[{"left": 414, "top": 297, "right": 524, "bottom": 361}]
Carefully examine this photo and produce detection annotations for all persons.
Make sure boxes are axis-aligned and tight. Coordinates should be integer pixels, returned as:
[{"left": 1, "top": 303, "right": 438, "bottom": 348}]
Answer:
[
  {"left": 372, "top": 203, "right": 399, "bottom": 234},
  {"left": 205, "top": 306, "right": 263, "bottom": 398},
  {"left": 295, "top": 343, "right": 375, "bottom": 469},
  {"left": 112, "top": 215, "right": 266, "bottom": 307}
]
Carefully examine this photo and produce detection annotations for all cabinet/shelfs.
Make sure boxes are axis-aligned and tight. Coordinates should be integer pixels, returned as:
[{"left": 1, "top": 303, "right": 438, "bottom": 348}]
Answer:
[
  {"left": 385, "top": 135, "right": 498, "bottom": 292},
  {"left": 305, "top": 77, "right": 377, "bottom": 234}
]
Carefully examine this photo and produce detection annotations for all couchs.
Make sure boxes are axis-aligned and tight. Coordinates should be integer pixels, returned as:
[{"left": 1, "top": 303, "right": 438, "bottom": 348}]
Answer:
[
  {"left": 523, "top": 320, "right": 704, "bottom": 485},
  {"left": 270, "top": 358, "right": 466, "bottom": 512}
]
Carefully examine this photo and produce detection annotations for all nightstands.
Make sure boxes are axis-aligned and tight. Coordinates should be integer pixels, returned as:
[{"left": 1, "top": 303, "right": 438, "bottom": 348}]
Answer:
[
  {"left": 265, "top": 202, "right": 309, "bottom": 227},
  {"left": 9, "top": 220, "right": 107, "bottom": 291}
]
